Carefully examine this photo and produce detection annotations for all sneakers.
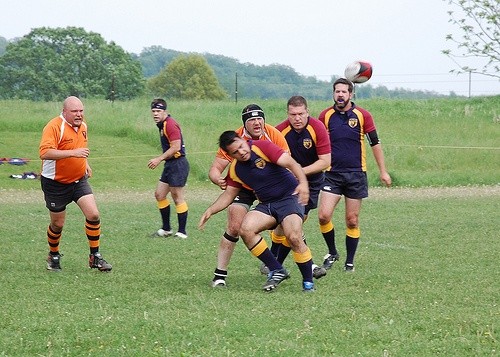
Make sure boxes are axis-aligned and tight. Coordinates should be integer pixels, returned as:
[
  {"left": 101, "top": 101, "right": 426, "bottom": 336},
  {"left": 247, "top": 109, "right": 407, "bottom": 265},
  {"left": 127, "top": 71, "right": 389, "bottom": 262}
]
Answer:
[
  {"left": 211, "top": 277, "right": 227, "bottom": 288},
  {"left": 344, "top": 263, "right": 354, "bottom": 271},
  {"left": 45, "top": 253, "right": 62, "bottom": 270},
  {"left": 320, "top": 254, "right": 339, "bottom": 269},
  {"left": 311, "top": 264, "right": 327, "bottom": 279},
  {"left": 303, "top": 281, "right": 315, "bottom": 293},
  {"left": 260, "top": 265, "right": 270, "bottom": 273},
  {"left": 261, "top": 269, "right": 288, "bottom": 290},
  {"left": 88, "top": 254, "right": 113, "bottom": 271}
]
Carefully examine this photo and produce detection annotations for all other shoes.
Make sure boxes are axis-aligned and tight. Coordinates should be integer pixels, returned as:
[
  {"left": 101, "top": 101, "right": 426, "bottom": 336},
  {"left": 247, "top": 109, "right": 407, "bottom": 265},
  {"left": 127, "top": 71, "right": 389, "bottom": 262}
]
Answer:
[
  {"left": 175, "top": 232, "right": 187, "bottom": 238},
  {"left": 157, "top": 229, "right": 172, "bottom": 237}
]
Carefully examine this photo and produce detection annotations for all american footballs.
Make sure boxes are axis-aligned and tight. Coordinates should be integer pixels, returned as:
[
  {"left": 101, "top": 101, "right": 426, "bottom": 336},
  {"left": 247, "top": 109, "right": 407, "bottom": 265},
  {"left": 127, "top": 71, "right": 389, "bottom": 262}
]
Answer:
[{"left": 344, "top": 60, "right": 372, "bottom": 84}]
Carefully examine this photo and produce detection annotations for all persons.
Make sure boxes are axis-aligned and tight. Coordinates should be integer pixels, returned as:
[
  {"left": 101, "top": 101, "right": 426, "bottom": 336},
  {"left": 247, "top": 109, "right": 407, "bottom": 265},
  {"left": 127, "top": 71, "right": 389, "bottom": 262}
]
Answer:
[
  {"left": 39, "top": 96, "right": 113, "bottom": 272},
  {"left": 198, "top": 131, "right": 316, "bottom": 291},
  {"left": 208, "top": 104, "right": 327, "bottom": 289},
  {"left": 260, "top": 96, "right": 332, "bottom": 275},
  {"left": 146, "top": 97, "right": 190, "bottom": 238},
  {"left": 316, "top": 78, "right": 392, "bottom": 271}
]
